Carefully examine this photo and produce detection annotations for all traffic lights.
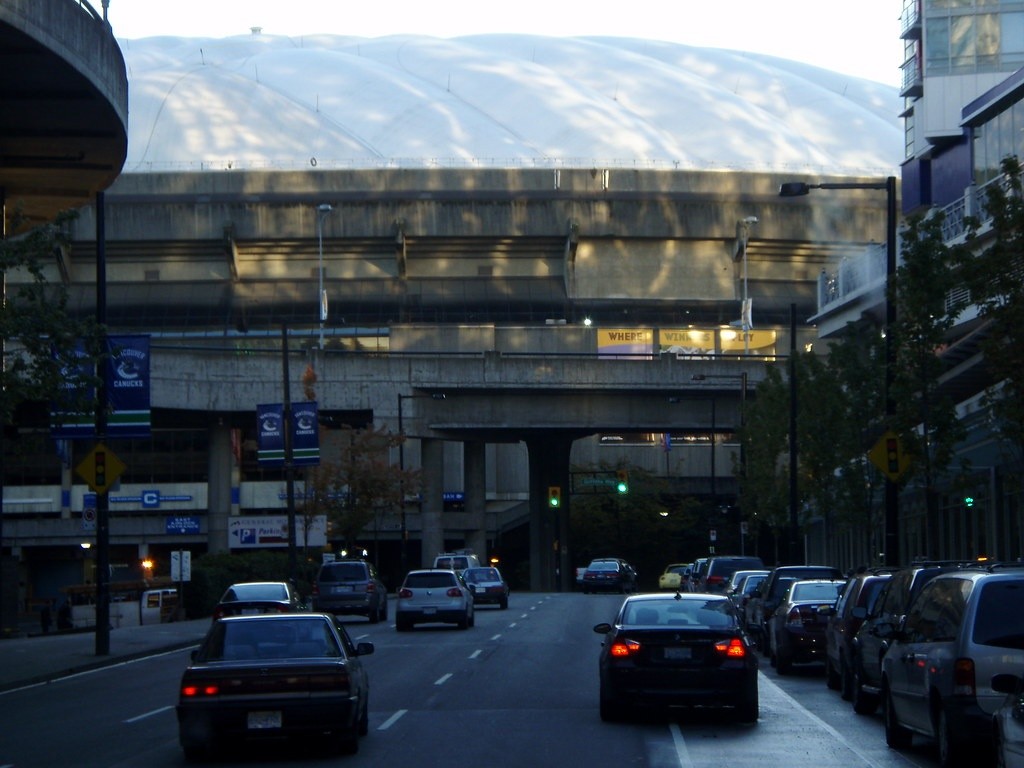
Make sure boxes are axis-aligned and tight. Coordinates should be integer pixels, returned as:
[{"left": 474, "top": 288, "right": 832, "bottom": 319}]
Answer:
[
  {"left": 964, "top": 495, "right": 976, "bottom": 508},
  {"left": 549, "top": 487, "right": 561, "bottom": 508},
  {"left": 618, "top": 466, "right": 631, "bottom": 494}
]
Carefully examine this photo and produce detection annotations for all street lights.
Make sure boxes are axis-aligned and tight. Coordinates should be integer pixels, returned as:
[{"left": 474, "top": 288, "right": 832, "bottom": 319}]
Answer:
[
  {"left": 692, "top": 369, "right": 759, "bottom": 556},
  {"left": 398, "top": 393, "right": 448, "bottom": 558},
  {"left": 776, "top": 173, "right": 903, "bottom": 569},
  {"left": 741, "top": 214, "right": 758, "bottom": 357},
  {"left": 317, "top": 204, "right": 333, "bottom": 350}
]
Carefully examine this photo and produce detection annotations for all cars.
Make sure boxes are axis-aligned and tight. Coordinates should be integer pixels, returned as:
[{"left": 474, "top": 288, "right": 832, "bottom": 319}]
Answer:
[
  {"left": 175, "top": 612, "right": 374, "bottom": 767},
  {"left": 457, "top": 565, "right": 511, "bottom": 609},
  {"left": 985, "top": 662, "right": 1024, "bottom": 768},
  {"left": 657, "top": 563, "right": 690, "bottom": 591},
  {"left": 681, "top": 553, "right": 988, "bottom": 715},
  {"left": 581, "top": 558, "right": 639, "bottom": 594},
  {"left": 593, "top": 591, "right": 766, "bottom": 725},
  {"left": 211, "top": 580, "right": 311, "bottom": 626},
  {"left": 395, "top": 568, "right": 476, "bottom": 630}
]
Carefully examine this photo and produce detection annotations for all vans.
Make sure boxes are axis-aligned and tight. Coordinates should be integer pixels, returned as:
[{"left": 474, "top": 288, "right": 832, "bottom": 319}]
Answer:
[{"left": 433, "top": 551, "right": 482, "bottom": 582}]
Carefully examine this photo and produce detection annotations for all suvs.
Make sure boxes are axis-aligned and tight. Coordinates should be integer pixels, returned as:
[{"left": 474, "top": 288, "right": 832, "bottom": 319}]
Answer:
[
  {"left": 311, "top": 559, "right": 389, "bottom": 623},
  {"left": 872, "top": 556, "right": 1024, "bottom": 768}
]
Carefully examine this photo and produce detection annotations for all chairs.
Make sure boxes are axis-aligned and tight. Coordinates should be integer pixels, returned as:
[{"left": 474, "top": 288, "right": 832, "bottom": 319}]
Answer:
[
  {"left": 225, "top": 646, "right": 253, "bottom": 656},
  {"left": 638, "top": 608, "right": 659, "bottom": 624},
  {"left": 697, "top": 612, "right": 725, "bottom": 625},
  {"left": 291, "top": 642, "right": 320, "bottom": 656}
]
